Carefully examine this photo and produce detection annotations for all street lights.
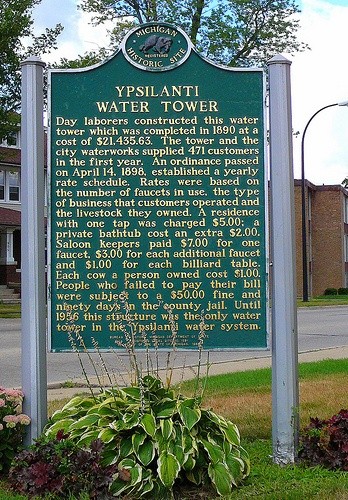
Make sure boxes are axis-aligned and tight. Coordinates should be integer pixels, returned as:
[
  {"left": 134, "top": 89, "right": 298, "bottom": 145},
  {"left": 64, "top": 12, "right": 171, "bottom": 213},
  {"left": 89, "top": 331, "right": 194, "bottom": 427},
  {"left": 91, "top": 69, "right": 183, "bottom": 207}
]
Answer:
[{"left": 301, "top": 100, "right": 348, "bottom": 302}]
